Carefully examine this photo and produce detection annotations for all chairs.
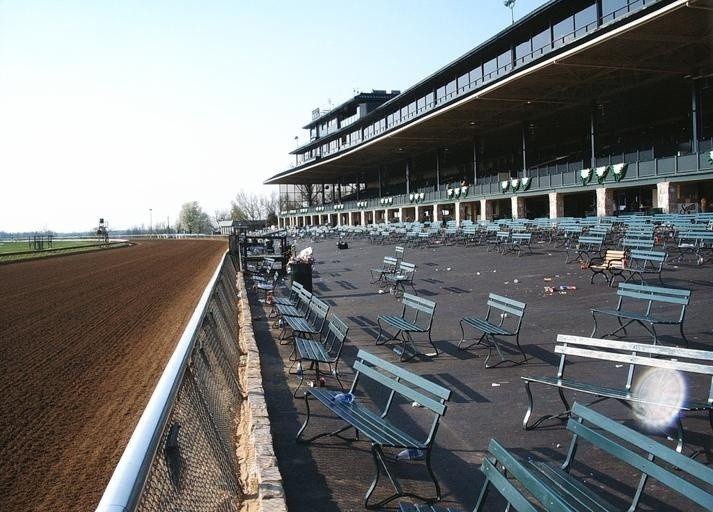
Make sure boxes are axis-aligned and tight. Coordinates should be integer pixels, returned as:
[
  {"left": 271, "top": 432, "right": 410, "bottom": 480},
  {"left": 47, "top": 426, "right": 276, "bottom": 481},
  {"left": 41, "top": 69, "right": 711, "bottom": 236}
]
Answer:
[
  {"left": 289, "top": 314, "right": 350, "bottom": 398},
  {"left": 295, "top": 347, "right": 453, "bottom": 510},
  {"left": 366, "top": 215, "right": 713, "bottom": 269},
  {"left": 587, "top": 249, "right": 627, "bottom": 288},
  {"left": 614, "top": 250, "right": 666, "bottom": 286},
  {"left": 369, "top": 247, "right": 420, "bottom": 298},
  {"left": 589, "top": 281, "right": 693, "bottom": 357},
  {"left": 399, "top": 437, "right": 571, "bottom": 512},
  {"left": 520, "top": 334, "right": 713, "bottom": 431},
  {"left": 456, "top": 291, "right": 529, "bottom": 369},
  {"left": 375, "top": 291, "right": 440, "bottom": 364},
  {"left": 251, "top": 256, "right": 331, "bottom": 346},
  {"left": 505, "top": 400, "right": 713, "bottom": 512},
  {"left": 253, "top": 223, "right": 366, "bottom": 242}
]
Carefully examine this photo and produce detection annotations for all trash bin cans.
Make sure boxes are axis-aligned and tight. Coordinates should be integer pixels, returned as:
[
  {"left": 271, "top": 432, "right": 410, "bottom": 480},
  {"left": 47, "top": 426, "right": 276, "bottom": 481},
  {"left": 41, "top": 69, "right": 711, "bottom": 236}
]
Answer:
[{"left": 290, "top": 263, "right": 312, "bottom": 298}]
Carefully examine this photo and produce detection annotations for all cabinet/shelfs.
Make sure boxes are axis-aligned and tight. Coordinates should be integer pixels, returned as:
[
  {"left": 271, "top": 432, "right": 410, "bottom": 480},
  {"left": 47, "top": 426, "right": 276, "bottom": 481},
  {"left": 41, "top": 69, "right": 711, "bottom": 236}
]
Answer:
[{"left": 239, "top": 234, "right": 286, "bottom": 277}]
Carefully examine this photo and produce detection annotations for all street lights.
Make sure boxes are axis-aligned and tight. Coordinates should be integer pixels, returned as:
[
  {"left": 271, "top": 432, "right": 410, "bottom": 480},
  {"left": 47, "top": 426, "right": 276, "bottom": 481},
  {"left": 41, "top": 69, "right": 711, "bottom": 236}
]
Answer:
[
  {"left": 504, "top": 0, "right": 516, "bottom": 24},
  {"left": 295, "top": 136, "right": 298, "bottom": 148}
]
